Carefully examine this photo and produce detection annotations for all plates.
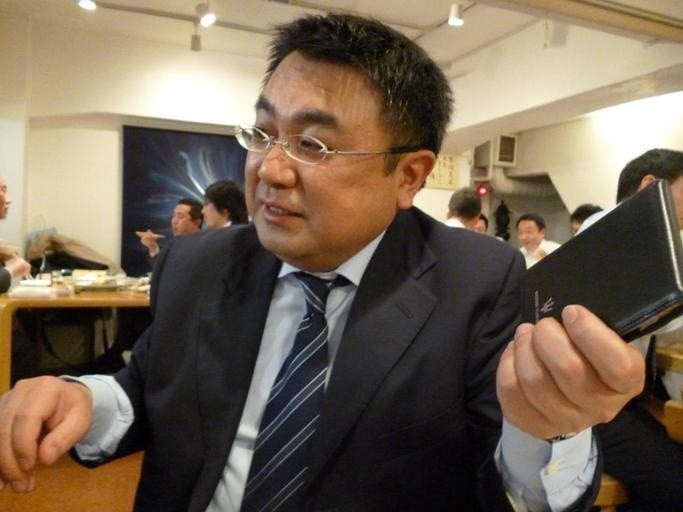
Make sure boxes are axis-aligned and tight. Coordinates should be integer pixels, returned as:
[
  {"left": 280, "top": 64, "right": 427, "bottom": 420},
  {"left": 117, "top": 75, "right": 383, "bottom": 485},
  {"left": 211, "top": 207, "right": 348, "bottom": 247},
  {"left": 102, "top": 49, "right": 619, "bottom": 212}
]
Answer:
[
  {"left": 20, "top": 279, "right": 52, "bottom": 287},
  {"left": 70, "top": 279, "right": 127, "bottom": 292}
]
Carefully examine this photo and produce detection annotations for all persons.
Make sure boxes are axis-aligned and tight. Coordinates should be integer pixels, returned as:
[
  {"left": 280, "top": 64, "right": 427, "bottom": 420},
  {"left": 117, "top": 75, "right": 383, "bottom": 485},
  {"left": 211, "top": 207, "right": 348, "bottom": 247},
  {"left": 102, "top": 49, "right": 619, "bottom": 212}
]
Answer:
[
  {"left": 573, "top": 145, "right": 683, "bottom": 404},
  {"left": 0, "top": 180, "right": 31, "bottom": 294},
  {"left": 200, "top": 178, "right": 249, "bottom": 228},
  {"left": 441, "top": 188, "right": 603, "bottom": 271},
  {"left": 0, "top": 11, "right": 645, "bottom": 512},
  {"left": 139, "top": 197, "right": 204, "bottom": 268}
]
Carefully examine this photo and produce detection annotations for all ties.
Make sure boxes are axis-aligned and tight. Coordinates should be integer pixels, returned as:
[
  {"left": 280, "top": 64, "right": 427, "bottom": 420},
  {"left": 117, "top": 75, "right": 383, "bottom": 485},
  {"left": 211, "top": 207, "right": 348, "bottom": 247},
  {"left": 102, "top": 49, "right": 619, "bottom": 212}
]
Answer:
[{"left": 238, "top": 272, "right": 353, "bottom": 511}]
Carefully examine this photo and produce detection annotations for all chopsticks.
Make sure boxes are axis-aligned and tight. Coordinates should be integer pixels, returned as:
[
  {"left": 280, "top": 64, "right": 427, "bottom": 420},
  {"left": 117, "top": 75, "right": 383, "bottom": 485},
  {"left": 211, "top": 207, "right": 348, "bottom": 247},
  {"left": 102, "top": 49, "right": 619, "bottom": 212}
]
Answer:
[
  {"left": 135, "top": 231, "right": 166, "bottom": 239},
  {"left": 12, "top": 253, "right": 34, "bottom": 281}
]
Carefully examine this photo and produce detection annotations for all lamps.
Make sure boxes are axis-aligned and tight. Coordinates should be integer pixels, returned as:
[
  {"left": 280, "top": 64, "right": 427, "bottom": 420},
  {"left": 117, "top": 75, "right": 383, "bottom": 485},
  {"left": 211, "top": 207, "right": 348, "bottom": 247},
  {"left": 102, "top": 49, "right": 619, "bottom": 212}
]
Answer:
[
  {"left": 449, "top": 2, "right": 464, "bottom": 27},
  {"left": 195, "top": 3, "right": 217, "bottom": 29}
]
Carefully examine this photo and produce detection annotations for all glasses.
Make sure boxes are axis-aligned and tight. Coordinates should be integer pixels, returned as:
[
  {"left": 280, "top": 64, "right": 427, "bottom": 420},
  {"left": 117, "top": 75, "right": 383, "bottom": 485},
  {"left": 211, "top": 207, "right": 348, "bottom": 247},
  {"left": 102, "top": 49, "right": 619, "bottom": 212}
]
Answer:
[{"left": 228, "top": 121, "right": 416, "bottom": 168}]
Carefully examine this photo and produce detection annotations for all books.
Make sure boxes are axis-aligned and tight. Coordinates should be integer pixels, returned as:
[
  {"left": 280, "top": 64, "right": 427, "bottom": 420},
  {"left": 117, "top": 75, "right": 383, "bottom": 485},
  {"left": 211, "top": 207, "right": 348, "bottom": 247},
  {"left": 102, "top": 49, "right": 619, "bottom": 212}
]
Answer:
[{"left": 520, "top": 177, "right": 682, "bottom": 342}]
